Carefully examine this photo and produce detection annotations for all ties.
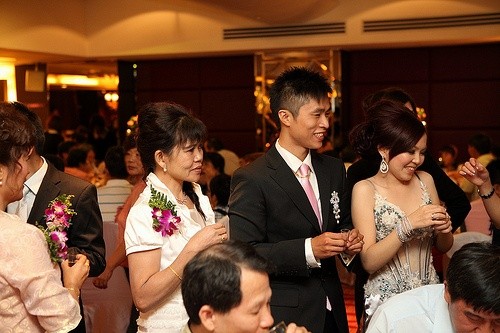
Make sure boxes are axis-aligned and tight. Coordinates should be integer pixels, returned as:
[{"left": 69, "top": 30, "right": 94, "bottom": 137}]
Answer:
[
  {"left": 299, "top": 163, "right": 322, "bottom": 232},
  {"left": 14, "top": 186, "right": 30, "bottom": 223}
]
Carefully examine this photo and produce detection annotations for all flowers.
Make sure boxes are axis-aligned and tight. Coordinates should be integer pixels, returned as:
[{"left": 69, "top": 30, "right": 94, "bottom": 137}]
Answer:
[
  {"left": 149, "top": 184, "right": 182, "bottom": 236},
  {"left": 43, "top": 194, "right": 77, "bottom": 232},
  {"left": 416, "top": 107, "right": 426, "bottom": 121},
  {"left": 35, "top": 221, "right": 69, "bottom": 267}
]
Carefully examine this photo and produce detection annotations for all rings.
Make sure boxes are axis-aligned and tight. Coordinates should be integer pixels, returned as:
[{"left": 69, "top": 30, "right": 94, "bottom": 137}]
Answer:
[
  {"left": 432, "top": 213, "right": 434, "bottom": 218},
  {"left": 221, "top": 235, "right": 225, "bottom": 240}
]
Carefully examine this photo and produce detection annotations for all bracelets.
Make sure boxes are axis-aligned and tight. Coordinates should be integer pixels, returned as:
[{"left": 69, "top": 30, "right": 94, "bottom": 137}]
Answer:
[
  {"left": 476, "top": 187, "right": 495, "bottom": 199},
  {"left": 394, "top": 218, "right": 416, "bottom": 243},
  {"left": 67, "top": 286, "right": 80, "bottom": 299},
  {"left": 168, "top": 266, "right": 183, "bottom": 282}
]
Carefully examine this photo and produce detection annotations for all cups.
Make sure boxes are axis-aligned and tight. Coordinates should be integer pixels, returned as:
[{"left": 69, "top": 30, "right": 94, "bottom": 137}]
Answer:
[
  {"left": 68, "top": 252, "right": 76, "bottom": 267},
  {"left": 435, "top": 202, "right": 445, "bottom": 207},
  {"left": 269, "top": 320, "right": 287, "bottom": 333},
  {"left": 342, "top": 229, "right": 350, "bottom": 246}
]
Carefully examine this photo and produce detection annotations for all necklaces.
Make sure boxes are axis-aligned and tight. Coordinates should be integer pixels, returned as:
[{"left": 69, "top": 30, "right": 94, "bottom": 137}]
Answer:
[{"left": 176, "top": 193, "right": 189, "bottom": 206}]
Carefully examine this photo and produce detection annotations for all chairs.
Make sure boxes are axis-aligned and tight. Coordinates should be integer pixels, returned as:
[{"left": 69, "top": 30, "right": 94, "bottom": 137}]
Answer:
[
  {"left": 79, "top": 222, "right": 133, "bottom": 333},
  {"left": 444, "top": 200, "right": 494, "bottom": 280}
]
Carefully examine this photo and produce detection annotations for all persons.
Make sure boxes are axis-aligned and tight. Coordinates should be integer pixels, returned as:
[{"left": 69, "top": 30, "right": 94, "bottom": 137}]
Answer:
[
  {"left": 0, "top": 113, "right": 91, "bottom": 333},
  {"left": 317, "top": 89, "right": 500, "bottom": 326},
  {"left": 178, "top": 238, "right": 312, "bottom": 333},
  {"left": 364, "top": 241, "right": 500, "bottom": 333},
  {"left": 93, "top": 136, "right": 148, "bottom": 333},
  {"left": 227, "top": 65, "right": 365, "bottom": 333},
  {"left": 349, "top": 102, "right": 453, "bottom": 333},
  {"left": 124, "top": 102, "right": 230, "bottom": 333},
  {"left": 39, "top": 109, "right": 129, "bottom": 188},
  {"left": 196, "top": 137, "right": 266, "bottom": 223},
  {"left": 4, "top": 101, "right": 107, "bottom": 333}
]
